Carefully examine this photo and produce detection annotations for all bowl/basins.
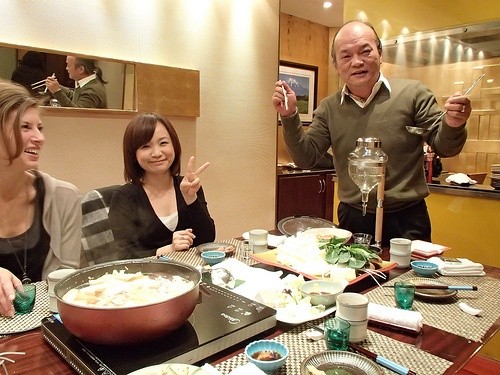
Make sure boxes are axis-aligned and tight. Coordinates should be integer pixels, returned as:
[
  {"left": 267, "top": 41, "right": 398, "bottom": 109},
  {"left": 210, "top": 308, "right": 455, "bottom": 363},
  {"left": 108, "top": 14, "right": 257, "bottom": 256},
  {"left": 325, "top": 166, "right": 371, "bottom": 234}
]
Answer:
[
  {"left": 245, "top": 341, "right": 289, "bottom": 374},
  {"left": 410, "top": 260, "right": 438, "bottom": 276},
  {"left": 201, "top": 251, "right": 226, "bottom": 265},
  {"left": 299, "top": 280, "right": 349, "bottom": 308}
]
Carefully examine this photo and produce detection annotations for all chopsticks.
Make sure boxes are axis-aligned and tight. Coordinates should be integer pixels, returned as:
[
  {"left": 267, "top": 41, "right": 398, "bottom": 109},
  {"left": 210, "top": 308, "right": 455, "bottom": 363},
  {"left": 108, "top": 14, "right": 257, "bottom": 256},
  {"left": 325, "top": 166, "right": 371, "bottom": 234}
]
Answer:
[
  {"left": 157, "top": 255, "right": 171, "bottom": 260},
  {"left": 382, "top": 285, "right": 478, "bottom": 291},
  {"left": 30, "top": 77, "right": 57, "bottom": 89},
  {"left": 279, "top": 79, "right": 289, "bottom": 110},
  {"left": 306, "top": 322, "right": 416, "bottom": 375}
]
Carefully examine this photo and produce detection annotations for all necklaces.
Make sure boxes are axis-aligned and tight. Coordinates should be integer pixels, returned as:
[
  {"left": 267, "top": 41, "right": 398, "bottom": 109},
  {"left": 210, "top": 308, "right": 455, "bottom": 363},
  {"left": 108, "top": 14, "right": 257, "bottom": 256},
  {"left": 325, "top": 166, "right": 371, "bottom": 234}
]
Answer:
[{"left": 3, "top": 171, "right": 30, "bottom": 285}]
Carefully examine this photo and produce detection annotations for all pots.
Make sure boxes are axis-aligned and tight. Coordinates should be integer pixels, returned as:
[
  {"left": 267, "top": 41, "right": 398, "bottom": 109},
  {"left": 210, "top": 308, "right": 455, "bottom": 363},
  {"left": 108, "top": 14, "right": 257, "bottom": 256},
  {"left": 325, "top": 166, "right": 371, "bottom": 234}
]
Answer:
[{"left": 53, "top": 260, "right": 201, "bottom": 344}]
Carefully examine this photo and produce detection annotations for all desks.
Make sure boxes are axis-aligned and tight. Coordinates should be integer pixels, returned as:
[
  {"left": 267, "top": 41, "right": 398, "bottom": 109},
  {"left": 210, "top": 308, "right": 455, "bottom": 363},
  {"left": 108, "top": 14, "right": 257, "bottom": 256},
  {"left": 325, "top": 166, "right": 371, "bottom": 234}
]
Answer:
[{"left": 0, "top": 229, "right": 500, "bottom": 375}]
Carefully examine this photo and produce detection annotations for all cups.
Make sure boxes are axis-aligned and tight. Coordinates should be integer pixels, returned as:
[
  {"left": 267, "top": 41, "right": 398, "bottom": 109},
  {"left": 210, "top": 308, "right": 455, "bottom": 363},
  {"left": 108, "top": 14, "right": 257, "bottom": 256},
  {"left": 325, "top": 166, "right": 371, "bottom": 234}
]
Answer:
[
  {"left": 324, "top": 317, "right": 351, "bottom": 351},
  {"left": 12, "top": 284, "right": 36, "bottom": 313},
  {"left": 47, "top": 269, "right": 76, "bottom": 313},
  {"left": 394, "top": 282, "right": 414, "bottom": 310},
  {"left": 353, "top": 233, "right": 372, "bottom": 246},
  {"left": 335, "top": 293, "right": 369, "bottom": 343},
  {"left": 389, "top": 238, "right": 411, "bottom": 268},
  {"left": 249, "top": 229, "right": 268, "bottom": 254}
]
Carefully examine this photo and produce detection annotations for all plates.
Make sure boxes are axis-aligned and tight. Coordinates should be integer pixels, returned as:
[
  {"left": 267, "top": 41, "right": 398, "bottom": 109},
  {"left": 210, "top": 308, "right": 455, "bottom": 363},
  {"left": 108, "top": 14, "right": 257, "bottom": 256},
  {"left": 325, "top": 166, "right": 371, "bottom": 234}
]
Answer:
[
  {"left": 126, "top": 363, "right": 204, "bottom": 375},
  {"left": 402, "top": 278, "right": 458, "bottom": 297},
  {"left": 300, "top": 350, "right": 382, "bottom": 375},
  {"left": 305, "top": 228, "right": 352, "bottom": 244},
  {"left": 198, "top": 242, "right": 235, "bottom": 253}
]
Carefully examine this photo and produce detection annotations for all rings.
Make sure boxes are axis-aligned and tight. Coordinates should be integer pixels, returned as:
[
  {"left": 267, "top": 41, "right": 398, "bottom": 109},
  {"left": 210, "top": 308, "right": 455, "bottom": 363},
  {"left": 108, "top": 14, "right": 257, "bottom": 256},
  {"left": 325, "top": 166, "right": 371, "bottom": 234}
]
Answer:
[
  {"left": 182, "top": 235, "right": 185, "bottom": 239},
  {"left": 461, "top": 106, "right": 465, "bottom": 112}
]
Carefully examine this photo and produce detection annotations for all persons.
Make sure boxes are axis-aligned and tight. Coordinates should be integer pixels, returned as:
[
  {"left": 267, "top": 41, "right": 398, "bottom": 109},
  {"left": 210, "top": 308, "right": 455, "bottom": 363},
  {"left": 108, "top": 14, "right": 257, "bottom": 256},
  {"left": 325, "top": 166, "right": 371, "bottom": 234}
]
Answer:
[
  {"left": 107, "top": 112, "right": 216, "bottom": 260},
  {"left": 0, "top": 81, "right": 83, "bottom": 317},
  {"left": 273, "top": 20, "right": 472, "bottom": 248},
  {"left": 9, "top": 51, "right": 110, "bottom": 108}
]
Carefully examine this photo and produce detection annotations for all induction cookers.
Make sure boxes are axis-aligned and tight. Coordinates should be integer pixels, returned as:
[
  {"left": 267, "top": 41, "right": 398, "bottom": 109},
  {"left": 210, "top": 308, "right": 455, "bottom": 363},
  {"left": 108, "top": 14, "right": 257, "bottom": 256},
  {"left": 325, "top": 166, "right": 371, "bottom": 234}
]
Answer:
[{"left": 41, "top": 279, "right": 277, "bottom": 375}]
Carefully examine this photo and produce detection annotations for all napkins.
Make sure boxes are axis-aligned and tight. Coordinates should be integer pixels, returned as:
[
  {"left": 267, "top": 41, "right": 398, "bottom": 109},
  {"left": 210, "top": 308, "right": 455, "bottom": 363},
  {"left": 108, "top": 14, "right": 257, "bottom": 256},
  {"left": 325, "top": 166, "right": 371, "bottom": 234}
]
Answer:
[
  {"left": 368, "top": 302, "right": 423, "bottom": 332},
  {"left": 427, "top": 257, "right": 487, "bottom": 277},
  {"left": 411, "top": 240, "right": 450, "bottom": 257}
]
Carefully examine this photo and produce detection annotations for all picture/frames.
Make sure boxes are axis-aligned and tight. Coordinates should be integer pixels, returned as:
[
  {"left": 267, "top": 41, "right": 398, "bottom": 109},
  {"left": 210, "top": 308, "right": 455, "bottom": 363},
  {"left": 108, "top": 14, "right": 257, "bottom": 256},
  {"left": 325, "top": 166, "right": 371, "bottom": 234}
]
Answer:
[{"left": 277, "top": 60, "right": 319, "bottom": 126}]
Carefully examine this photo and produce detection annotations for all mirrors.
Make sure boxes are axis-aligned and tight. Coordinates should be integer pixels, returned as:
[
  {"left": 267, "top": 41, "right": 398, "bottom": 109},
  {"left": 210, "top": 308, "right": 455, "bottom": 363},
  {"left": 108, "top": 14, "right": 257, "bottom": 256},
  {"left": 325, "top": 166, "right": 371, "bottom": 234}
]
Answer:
[{"left": 0, "top": 46, "right": 135, "bottom": 110}]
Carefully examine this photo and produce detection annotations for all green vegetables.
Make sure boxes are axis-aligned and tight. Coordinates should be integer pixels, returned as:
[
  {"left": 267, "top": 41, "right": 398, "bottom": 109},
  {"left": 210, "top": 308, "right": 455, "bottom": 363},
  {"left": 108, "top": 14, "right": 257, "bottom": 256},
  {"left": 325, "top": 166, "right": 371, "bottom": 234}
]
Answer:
[{"left": 318, "top": 236, "right": 378, "bottom": 270}]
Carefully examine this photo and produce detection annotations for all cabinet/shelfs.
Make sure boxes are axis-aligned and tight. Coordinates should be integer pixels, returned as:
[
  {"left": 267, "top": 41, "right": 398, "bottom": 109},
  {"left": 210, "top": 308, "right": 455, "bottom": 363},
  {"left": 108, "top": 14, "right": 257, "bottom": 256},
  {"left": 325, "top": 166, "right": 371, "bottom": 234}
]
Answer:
[{"left": 277, "top": 170, "right": 334, "bottom": 221}]
